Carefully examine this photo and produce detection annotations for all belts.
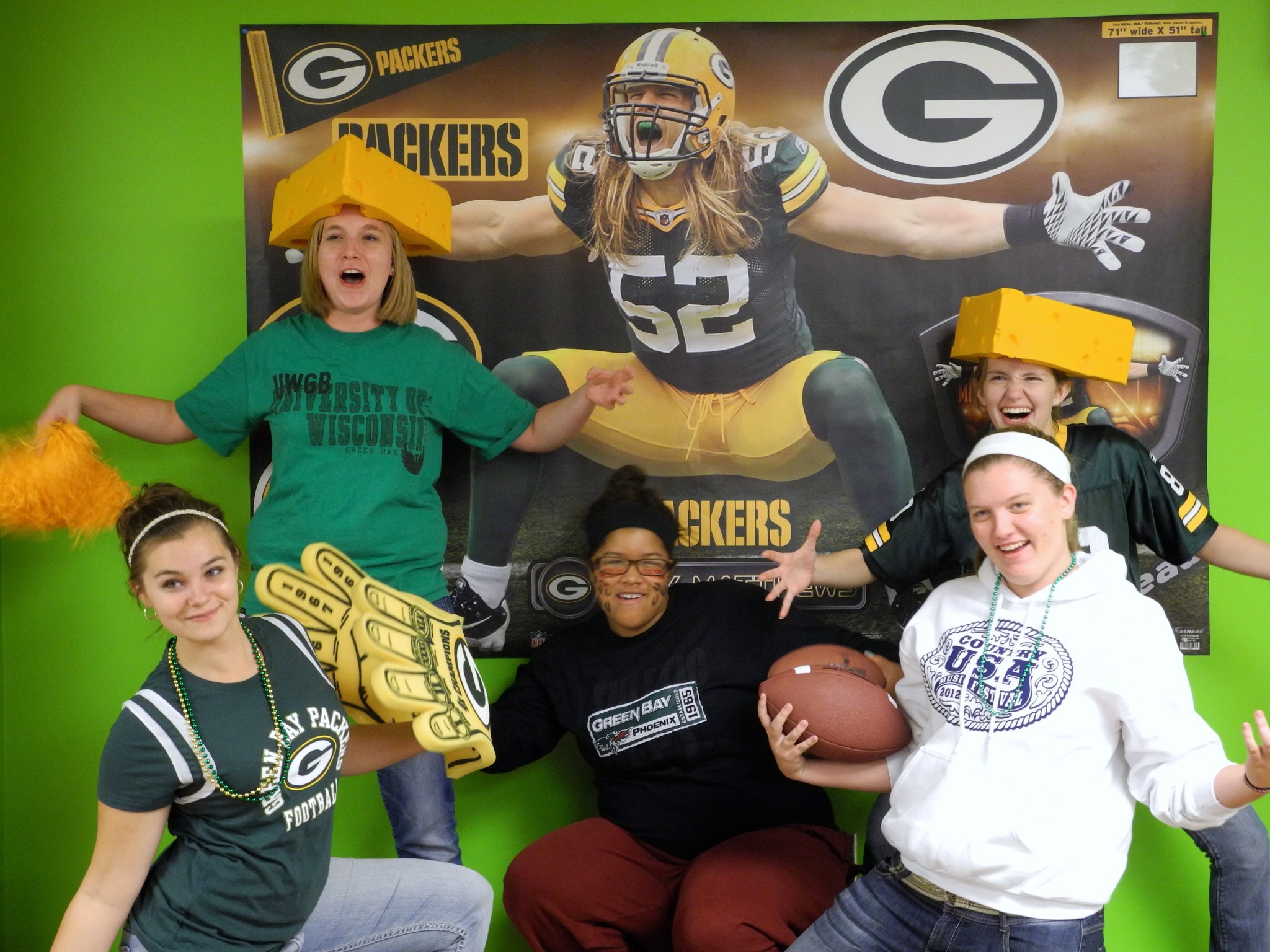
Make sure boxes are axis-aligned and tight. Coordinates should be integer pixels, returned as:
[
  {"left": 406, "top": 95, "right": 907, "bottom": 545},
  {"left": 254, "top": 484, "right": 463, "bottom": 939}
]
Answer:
[{"left": 889, "top": 860, "right": 1018, "bottom": 918}]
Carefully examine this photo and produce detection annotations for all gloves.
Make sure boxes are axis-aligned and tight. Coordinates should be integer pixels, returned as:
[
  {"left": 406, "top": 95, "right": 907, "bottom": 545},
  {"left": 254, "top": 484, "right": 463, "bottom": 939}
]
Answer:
[
  {"left": 1031, "top": 171, "right": 1151, "bottom": 271},
  {"left": 1158, "top": 355, "right": 1189, "bottom": 383},
  {"left": 933, "top": 362, "right": 962, "bottom": 386},
  {"left": 257, "top": 542, "right": 498, "bottom": 781}
]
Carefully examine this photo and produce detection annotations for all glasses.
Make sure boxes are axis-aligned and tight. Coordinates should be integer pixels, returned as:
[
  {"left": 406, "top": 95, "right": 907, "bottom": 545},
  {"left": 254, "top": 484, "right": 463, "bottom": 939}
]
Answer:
[{"left": 594, "top": 556, "right": 672, "bottom": 576}]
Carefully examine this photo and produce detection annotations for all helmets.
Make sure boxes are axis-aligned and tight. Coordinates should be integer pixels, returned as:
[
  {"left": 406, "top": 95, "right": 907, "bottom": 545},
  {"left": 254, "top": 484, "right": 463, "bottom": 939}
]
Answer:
[{"left": 599, "top": 28, "right": 735, "bottom": 179}]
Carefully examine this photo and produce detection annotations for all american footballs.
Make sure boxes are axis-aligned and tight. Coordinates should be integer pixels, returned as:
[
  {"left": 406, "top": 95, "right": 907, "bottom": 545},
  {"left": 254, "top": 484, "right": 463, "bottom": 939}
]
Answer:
[
  {"left": 760, "top": 643, "right": 886, "bottom": 687},
  {"left": 761, "top": 666, "right": 914, "bottom": 760}
]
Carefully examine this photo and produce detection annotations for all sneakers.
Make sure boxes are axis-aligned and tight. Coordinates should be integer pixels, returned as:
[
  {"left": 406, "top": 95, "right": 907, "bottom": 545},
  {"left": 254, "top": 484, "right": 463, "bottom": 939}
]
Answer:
[
  {"left": 448, "top": 577, "right": 511, "bottom": 653},
  {"left": 885, "top": 580, "right": 930, "bottom": 629}
]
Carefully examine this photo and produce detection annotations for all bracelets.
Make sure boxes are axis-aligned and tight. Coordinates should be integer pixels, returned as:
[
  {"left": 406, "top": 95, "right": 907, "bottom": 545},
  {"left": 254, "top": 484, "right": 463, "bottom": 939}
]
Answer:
[{"left": 1243, "top": 772, "right": 1270, "bottom": 793}]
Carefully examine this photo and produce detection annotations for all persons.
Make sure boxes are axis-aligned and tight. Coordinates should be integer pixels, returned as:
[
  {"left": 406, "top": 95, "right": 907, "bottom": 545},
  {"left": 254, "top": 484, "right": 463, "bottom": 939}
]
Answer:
[
  {"left": 757, "top": 356, "right": 1270, "bottom": 630},
  {"left": 758, "top": 424, "right": 1270, "bottom": 952},
  {"left": 436, "top": 27, "right": 1150, "bottom": 655},
  {"left": 488, "top": 466, "right": 904, "bottom": 952},
  {"left": 51, "top": 481, "right": 494, "bottom": 952},
  {"left": 36, "top": 205, "right": 632, "bottom": 869},
  {"left": 932, "top": 355, "right": 1190, "bottom": 426}
]
